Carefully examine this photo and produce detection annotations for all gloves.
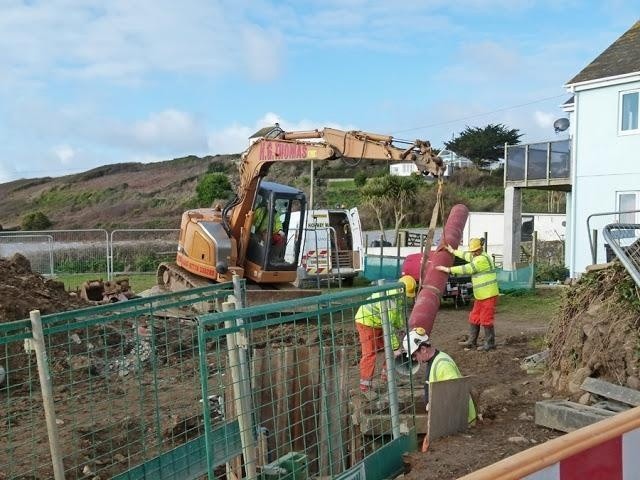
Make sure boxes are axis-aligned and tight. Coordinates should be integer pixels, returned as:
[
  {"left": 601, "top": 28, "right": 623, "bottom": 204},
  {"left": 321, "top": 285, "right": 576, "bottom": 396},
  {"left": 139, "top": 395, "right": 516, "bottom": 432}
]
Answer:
[
  {"left": 435, "top": 265, "right": 450, "bottom": 273},
  {"left": 445, "top": 243, "right": 454, "bottom": 253},
  {"left": 394, "top": 347, "right": 402, "bottom": 357}
]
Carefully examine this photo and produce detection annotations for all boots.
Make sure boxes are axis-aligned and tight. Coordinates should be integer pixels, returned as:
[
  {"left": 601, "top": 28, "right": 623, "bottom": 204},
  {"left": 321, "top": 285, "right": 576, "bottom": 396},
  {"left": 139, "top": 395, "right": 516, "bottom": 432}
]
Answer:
[
  {"left": 458, "top": 322, "right": 480, "bottom": 347},
  {"left": 360, "top": 384, "right": 369, "bottom": 402},
  {"left": 477, "top": 326, "right": 496, "bottom": 351},
  {"left": 381, "top": 368, "right": 410, "bottom": 386}
]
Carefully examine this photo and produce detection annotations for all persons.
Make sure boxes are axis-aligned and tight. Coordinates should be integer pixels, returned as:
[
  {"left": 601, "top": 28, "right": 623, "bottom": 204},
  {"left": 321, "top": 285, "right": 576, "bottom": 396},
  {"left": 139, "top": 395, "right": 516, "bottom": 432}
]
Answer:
[
  {"left": 254, "top": 207, "right": 286, "bottom": 249},
  {"left": 435, "top": 238, "right": 499, "bottom": 351},
  {"left": 403, "top": 327, "right": 477, "bottom": 452},
  {"left": 354, "top": 274, "right": 417, "bottom": 401}
]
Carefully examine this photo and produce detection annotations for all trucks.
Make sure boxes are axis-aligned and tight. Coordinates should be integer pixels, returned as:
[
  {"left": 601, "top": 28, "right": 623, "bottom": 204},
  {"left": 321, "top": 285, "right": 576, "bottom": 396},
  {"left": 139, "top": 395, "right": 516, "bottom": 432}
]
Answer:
[{"left": 279, "top": 208, "right": 364, "bottom": 287}]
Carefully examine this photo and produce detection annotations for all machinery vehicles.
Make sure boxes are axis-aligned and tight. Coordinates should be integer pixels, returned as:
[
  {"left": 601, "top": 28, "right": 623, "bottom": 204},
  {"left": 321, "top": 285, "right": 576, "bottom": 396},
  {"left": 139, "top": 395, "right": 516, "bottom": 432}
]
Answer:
[{"left": 155, "top": 127, "right": 448, "bottom": 315}]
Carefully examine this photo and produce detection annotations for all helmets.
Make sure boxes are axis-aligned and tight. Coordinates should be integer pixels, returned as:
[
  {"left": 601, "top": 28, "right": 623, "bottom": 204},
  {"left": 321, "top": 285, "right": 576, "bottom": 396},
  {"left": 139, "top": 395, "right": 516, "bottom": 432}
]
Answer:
[
  {"left": 403, "top": 330, "right": 429, "bottom": 358},
  {"left": 398, "top": 275, "right": 416, "bottom": 298},
  {"left": 467, "top": 237, "right": 482, "bottom": 251}
]
